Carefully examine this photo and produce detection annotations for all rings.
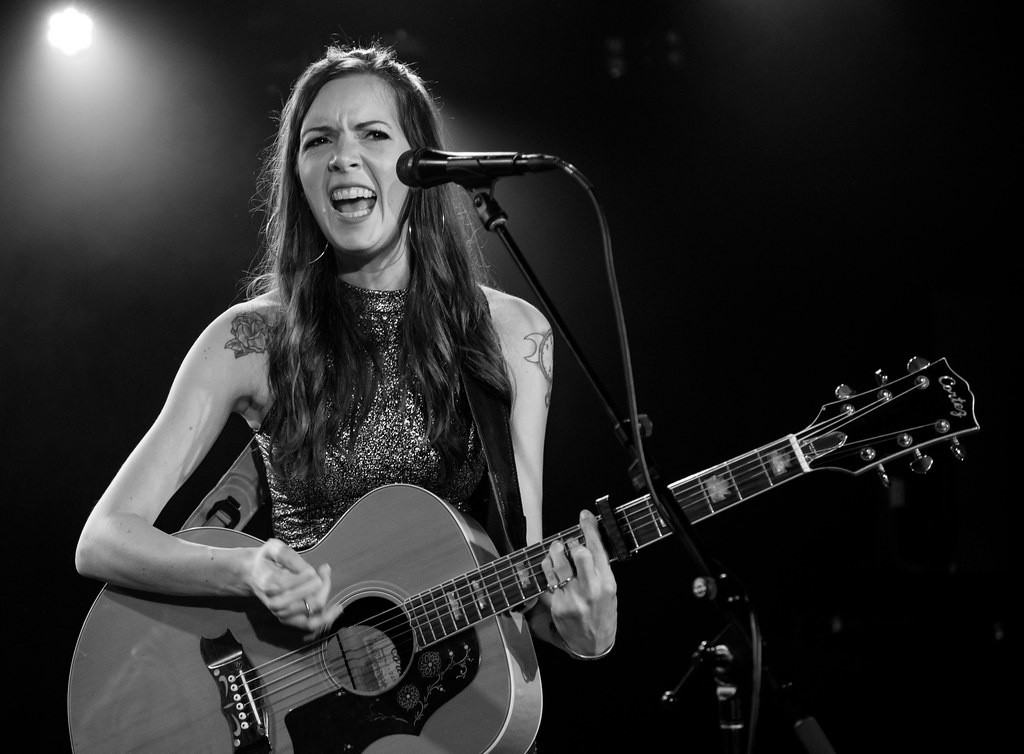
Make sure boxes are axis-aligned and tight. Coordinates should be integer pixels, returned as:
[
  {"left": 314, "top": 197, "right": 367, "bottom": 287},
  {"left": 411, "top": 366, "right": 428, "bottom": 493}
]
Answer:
[
  {"left": 556, "top": 575, "right": 574, "bottom": 588},
  {"left": 324, "top": 624, "right": 330, "bottom": 632},
  {"left": 305, "top": 598, "right": 312, "bottom": 616},
  {"left": 545, "top": 583, "right": 556, "bottom": 594}
]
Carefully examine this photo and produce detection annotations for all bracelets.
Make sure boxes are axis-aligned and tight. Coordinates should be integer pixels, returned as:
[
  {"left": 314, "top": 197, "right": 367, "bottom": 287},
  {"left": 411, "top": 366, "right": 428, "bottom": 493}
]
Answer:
[{"left": 562, "top": 642, "right": 616, "bottom": 660}]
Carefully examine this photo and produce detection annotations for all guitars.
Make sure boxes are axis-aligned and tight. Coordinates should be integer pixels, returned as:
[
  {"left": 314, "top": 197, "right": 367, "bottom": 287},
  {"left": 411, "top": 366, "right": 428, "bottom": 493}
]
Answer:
[{"left": 63, "top": 349, "right": 984, "bottom": 752}]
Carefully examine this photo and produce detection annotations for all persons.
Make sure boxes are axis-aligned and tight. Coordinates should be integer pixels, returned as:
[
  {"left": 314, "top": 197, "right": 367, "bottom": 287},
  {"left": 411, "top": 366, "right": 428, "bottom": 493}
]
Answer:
[{"left": 73, "top": 37, "right": 617, "bottom": 753}]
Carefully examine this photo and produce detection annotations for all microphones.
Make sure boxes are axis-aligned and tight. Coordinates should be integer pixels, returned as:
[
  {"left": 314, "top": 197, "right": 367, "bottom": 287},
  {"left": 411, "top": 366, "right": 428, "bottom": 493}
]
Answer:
[{"left": 396, "top": 147, "right": 562, "bottom": 190}]
